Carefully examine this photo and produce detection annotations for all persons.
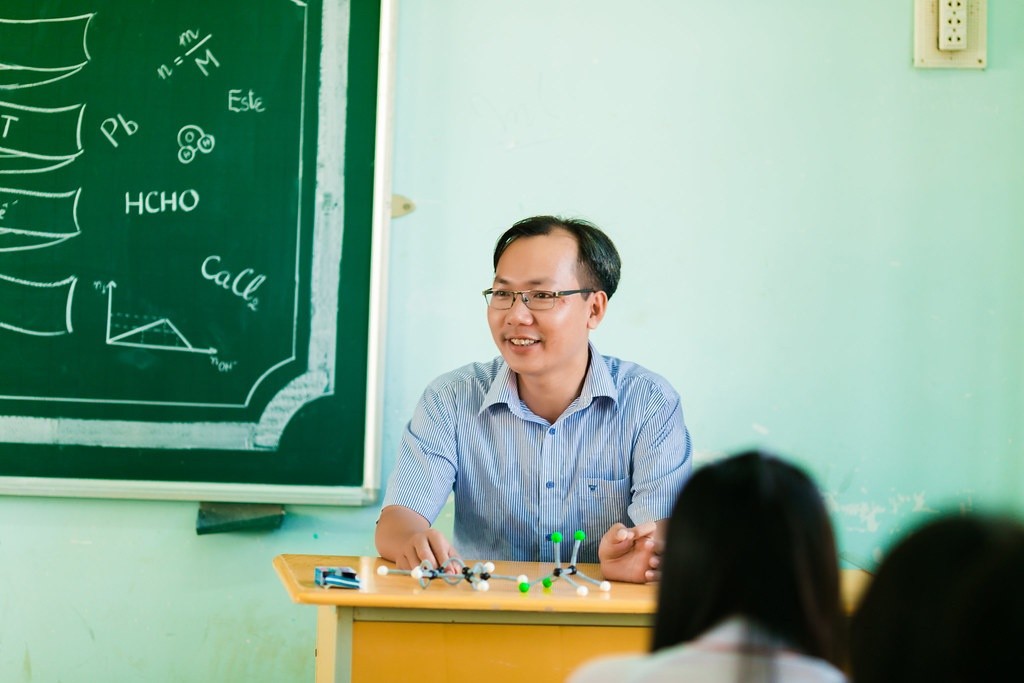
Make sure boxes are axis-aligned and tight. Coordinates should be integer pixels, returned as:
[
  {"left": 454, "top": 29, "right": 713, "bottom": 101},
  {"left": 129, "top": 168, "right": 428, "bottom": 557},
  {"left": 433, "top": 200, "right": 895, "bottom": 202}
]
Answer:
[
  {"left": 564, "top": 451, "right": 849, "bottom": 683},
  {"left": 375, "top": 218, "right": 694, "bottom": 585},
  {"left": 842, "top": 510, "right": 1024, "bottom": 683}
]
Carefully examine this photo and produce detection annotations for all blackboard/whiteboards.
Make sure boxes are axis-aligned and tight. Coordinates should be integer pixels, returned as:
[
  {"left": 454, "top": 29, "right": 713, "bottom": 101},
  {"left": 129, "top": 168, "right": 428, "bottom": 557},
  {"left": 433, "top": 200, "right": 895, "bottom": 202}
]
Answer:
[{"left": 0, "top": 0, "right": 398, "bottom": 508}]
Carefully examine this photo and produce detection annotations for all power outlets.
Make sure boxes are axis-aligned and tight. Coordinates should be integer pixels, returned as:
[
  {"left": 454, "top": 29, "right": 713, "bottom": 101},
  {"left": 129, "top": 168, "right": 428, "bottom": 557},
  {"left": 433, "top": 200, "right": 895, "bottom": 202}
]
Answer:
[{"left": 937, "top": 0, "right": 968, "bottom": 50}]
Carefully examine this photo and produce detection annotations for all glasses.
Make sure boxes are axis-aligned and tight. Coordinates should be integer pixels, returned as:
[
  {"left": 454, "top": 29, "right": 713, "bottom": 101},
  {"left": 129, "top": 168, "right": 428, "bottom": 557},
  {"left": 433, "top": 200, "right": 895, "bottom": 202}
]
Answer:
[{"left": 482, "top": 287, "right": 596, "bottom": 311}]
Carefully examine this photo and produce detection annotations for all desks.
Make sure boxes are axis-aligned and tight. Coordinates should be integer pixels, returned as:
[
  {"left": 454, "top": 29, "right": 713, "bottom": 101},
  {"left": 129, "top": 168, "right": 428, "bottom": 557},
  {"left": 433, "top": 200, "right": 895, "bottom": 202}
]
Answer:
[{"left": 270, "top": 552, "right": 874, "bottom": 683}]
